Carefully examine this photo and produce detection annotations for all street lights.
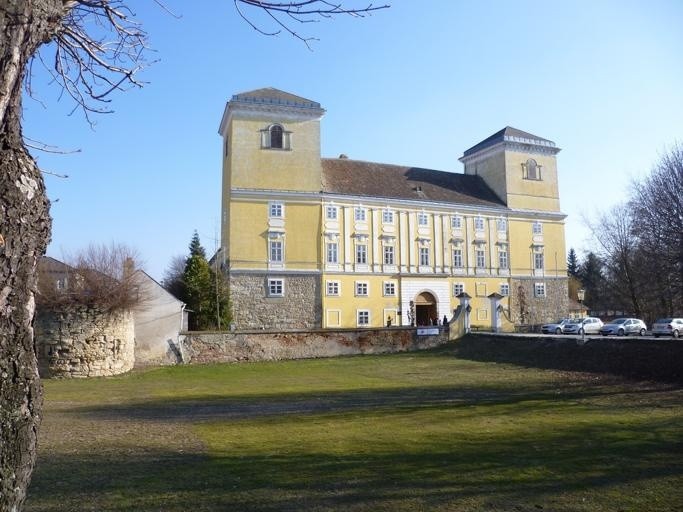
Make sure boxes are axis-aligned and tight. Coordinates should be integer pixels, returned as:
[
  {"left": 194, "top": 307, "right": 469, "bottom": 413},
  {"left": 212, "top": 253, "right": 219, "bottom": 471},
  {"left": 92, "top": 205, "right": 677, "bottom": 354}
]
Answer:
[{"left": 577, "top": 287, "right": 585, "bottom": 341}]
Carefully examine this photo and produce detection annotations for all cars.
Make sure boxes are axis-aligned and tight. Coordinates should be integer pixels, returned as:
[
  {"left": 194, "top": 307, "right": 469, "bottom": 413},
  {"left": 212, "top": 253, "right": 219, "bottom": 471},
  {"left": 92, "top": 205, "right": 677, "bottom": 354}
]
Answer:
[
  {"left": 598, "top": 317, "right": 648, "bottom": 337},
  {"left": 540, "top": 319, "right": 575, "bottom": 335},
  {"left": 562, "top": 317, "right": 604, "bottom": 335},
  {"left": 651, "top": 317, "right": 682, "bottom": 338}
]
